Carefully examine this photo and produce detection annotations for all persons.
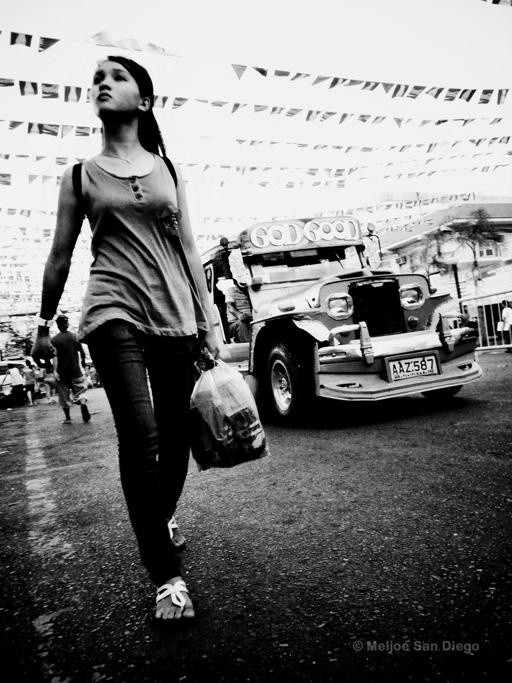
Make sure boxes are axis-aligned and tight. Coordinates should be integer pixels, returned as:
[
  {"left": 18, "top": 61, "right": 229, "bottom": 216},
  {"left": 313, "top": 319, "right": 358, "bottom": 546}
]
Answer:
[
  {"left": 225, "top": 271, "right": 252, "bottom": 343},
  {"left": 42, "top": 358, "right": 60, "bottom": 405},
  {"left": 501, "top": 300, "right": 512, "bottom": 353},
  {"left": 31, "top": 55, "right": 225, "bottom": 626},
  {"left": 1, "top": 363, "right": 24, "bottom": 411},
  {"left": 50, "top": 316, "right": 91, "bottom": 424},
  {"left": 21, "top": 360, "right": 36, "bottom": 407}
]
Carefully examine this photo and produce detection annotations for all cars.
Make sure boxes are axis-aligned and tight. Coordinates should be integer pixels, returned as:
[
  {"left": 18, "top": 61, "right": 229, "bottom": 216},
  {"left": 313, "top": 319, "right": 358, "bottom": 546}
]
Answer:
[
  {"left": 196, "top": 214, "right": 488, "bottom": 418},
  {"left": 0, "top": 354, "right": 45, "bottom": 404}
]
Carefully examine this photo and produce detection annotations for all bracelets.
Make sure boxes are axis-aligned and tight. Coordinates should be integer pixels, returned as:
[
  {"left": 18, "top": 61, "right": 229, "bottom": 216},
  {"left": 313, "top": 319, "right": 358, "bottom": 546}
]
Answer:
[{"left": 37, "top": 316, "right": 53, "bottom": 328}]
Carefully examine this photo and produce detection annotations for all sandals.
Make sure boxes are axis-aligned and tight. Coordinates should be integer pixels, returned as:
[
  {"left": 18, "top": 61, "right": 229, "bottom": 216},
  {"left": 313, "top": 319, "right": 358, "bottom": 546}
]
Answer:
[
  {"left": 155, "top": 577, "right": 194, "bottom": 619},
  {"left": 167, "top": 515, "right": 186, "bottom": 548}
]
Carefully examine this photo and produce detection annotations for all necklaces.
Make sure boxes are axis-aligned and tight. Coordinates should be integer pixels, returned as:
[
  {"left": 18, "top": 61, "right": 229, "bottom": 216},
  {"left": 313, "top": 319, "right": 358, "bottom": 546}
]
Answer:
[{"left": 105, "top": 153, "right": 136, "bottom": 163}]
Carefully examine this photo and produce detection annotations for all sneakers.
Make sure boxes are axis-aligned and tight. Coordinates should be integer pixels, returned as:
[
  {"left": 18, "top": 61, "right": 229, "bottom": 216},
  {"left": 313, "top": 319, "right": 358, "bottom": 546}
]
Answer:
[
  {"left": 81, "top": 404, "right": 89, "bottom": 423},
  {"left": 63, "top": 418, "right": 71, "bottom": 423}
]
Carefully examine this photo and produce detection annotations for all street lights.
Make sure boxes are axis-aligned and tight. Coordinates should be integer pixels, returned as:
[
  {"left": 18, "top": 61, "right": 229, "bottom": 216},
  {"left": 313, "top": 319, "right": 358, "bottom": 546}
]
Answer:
[{"left": 445, "top": 256, "right": 464, "bottom": 315}]
